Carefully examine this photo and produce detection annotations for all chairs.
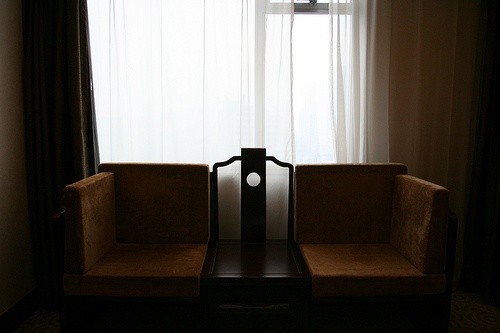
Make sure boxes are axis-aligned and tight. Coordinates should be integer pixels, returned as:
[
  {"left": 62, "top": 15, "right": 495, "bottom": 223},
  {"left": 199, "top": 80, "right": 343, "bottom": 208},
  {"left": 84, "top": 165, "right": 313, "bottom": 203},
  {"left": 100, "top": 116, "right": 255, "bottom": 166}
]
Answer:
[
  {"left": 60, "top": 162, "right": 213, "bottom": 333},
  {"left": 199, "top": 147, "right": 313, "bottom": 332},
  {"left": 293, "top": 159, "right": 459, "bottom": 333}
]
